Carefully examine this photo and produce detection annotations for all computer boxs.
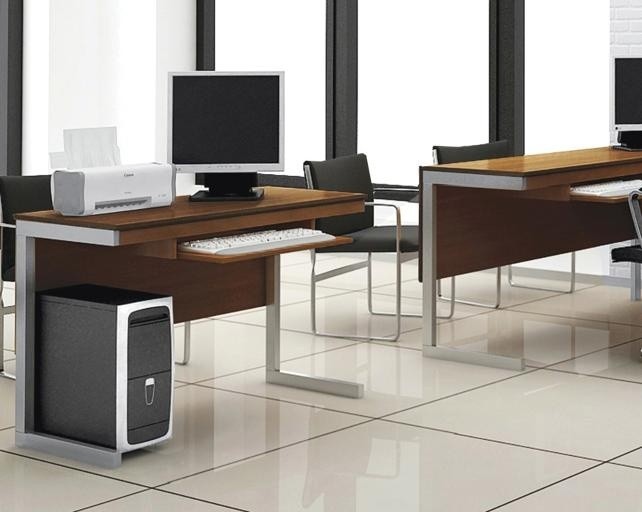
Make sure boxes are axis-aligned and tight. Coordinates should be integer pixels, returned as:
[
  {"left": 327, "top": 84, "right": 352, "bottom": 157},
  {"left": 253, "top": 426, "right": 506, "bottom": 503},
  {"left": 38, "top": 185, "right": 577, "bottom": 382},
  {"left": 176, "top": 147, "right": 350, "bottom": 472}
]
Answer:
[{"left": 33, "top": 282, "right": 175, "bottom": 454}]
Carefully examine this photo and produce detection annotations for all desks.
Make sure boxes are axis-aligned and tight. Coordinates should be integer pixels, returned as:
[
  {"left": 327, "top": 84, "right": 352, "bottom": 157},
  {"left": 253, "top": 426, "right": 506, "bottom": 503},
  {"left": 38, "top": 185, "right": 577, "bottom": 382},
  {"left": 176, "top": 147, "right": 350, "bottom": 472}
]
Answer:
[
  {"left": 419, "top": 145, "right": 642, "bottom": 372},
  {"left": 12, "top": 184, "right": 368, "bottom": 468}
]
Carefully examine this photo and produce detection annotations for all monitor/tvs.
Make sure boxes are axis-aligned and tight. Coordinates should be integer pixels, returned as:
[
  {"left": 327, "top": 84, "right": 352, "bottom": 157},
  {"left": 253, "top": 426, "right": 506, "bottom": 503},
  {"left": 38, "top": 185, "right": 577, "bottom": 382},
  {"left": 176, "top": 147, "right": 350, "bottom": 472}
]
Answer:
[
  {"left": 166, "top": 70, "right": 286, "bottom": 200},
  {"left": 608, "top": 54, "right": 642, "bottom": 153}
]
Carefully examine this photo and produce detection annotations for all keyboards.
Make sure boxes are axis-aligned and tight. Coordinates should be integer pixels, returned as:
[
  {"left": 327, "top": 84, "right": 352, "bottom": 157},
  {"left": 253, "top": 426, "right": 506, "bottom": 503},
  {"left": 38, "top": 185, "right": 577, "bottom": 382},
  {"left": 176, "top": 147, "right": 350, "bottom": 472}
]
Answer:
[
  {"left": 571, "top": 178, "right": 642, "bottom": 197},
  {"left": 177, "top": 226, "right": 337, "bottom": 256}
]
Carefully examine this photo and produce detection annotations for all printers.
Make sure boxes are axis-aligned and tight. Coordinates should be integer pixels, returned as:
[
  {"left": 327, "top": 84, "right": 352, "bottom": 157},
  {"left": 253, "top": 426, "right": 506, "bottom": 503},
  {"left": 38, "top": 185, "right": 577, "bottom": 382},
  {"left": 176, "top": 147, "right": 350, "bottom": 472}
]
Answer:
[{"left": 49, "top": 147, "right": 176, "bottom": 217}]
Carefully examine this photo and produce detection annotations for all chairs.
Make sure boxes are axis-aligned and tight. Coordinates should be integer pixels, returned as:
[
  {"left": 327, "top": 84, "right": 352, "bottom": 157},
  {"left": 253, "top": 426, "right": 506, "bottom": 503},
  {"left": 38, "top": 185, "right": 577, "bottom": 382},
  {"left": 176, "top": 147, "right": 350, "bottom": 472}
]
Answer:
[
  {"left": 303, "top": 153, "right": 455, "bottom": 342},
  {"left": 0, "top": 174, "right": 55, "bottom": 380},
  {"left": 611, "top": 187, "right": 642, "bottom": 300},
  {"left": 432, "top": 139, "right": 575, "bottom": 309}
]
